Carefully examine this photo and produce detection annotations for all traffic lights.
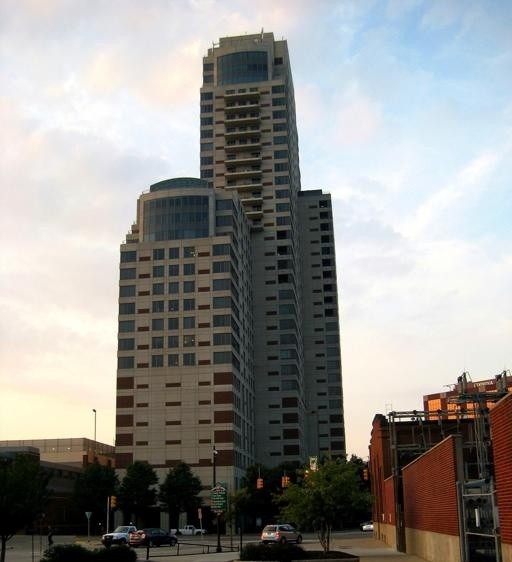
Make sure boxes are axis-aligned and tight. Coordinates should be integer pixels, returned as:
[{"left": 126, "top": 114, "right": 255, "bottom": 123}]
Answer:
[
  {"left": 257, "top": 478, "right": 264, "bottom": 490},
  {"left": 110, "top": 496, "right": 117, "bottom": 510},
  {"left": 363, "top": 468, "right": 369, "bottom": 481},
  {"left": 281, "top": 476, "right": 291, "bottom": 488},
  {"left": 305, "top": 470, "right": 309, "bottom": 480}
]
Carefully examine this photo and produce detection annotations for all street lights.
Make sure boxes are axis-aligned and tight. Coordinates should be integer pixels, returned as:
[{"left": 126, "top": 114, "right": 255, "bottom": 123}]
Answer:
[
  {"left": 212, "top": 447, "right": 218, "bottom": 488},
  {"left": 92, "top": 409, "right": 96, "bottom": 453}
]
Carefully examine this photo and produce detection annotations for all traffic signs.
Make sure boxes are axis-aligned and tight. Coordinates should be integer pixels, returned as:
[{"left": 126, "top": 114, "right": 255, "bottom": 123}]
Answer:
[{"left": 211, "top": 488, "right": 226, "bottom": 512}]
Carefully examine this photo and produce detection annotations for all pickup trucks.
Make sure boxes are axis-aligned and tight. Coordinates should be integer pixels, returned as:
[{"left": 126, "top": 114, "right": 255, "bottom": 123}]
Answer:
[
  {"left": 171, "top": 525, "right": 207, "bottom": 536},
  {"left": 101, "top": 526, "right": 137, "bottom": 548}
]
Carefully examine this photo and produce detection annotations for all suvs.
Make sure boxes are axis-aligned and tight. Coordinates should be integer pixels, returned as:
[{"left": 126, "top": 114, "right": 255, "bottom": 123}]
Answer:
[{"left": 261, "top": 524, "right": 303, "bottom": 544}]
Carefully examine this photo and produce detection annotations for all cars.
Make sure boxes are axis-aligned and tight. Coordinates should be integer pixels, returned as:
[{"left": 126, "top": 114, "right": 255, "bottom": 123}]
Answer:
[
  {"left": 127, "top": 528, "right": 177, "bottom": 548},
  {"left": 362, "top": 520, "right": 374, "bottom": 531}
]
